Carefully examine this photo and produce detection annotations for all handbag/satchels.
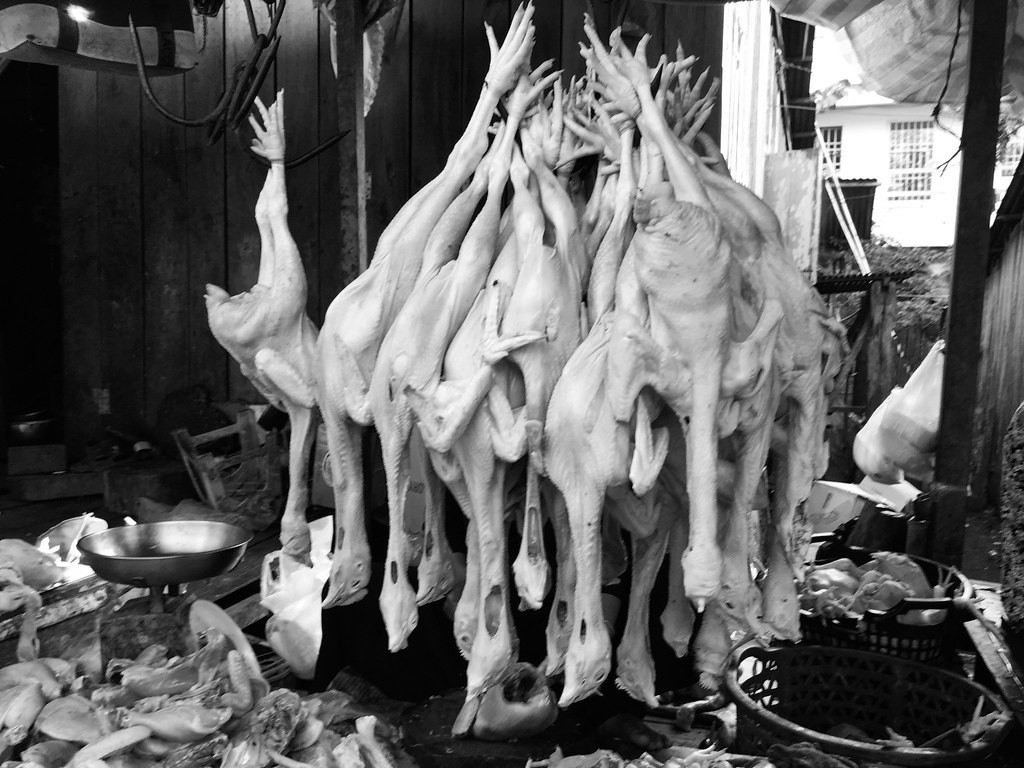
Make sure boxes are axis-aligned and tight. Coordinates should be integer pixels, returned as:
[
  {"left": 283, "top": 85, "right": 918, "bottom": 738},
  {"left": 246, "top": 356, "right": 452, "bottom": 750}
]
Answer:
[
  {"left": 850, "top": 385, "right": 913, "bottom": 482},
  {"left": 879, "top": 340, "right": 950, "bottom": 484}
]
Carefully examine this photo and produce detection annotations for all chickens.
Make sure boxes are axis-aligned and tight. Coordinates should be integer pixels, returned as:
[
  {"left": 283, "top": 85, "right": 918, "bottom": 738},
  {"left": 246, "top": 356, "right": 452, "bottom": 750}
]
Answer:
[{"left": 1, "top": 0, "right": 1023, "bottom": 765}]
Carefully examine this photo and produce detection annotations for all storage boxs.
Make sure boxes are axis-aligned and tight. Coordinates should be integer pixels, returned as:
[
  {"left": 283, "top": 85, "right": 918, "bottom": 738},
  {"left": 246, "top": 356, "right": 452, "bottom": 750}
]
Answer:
[
  {"left": 800, "top": 551, "right": 966, "bottom": 667},
  {"left": 722, "top": 639, "right": 1013, "bottom": 768}
]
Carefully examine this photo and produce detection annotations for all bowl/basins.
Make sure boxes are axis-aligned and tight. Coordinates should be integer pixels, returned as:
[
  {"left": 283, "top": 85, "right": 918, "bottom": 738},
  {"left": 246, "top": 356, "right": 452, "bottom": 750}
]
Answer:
[
  {"left": 8, "top": 416, "right": 55, "bottom": 445},
  {"left": 10, "top": 405, "right": 49, "bottom": 422},
  {"left": 76, "top": 519, "right": 255, "bottom": 587}
]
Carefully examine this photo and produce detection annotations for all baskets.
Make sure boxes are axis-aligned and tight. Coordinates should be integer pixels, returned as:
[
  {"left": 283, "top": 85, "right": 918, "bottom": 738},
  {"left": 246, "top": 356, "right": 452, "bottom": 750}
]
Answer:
[
  {"left": 798, "top": 543, "right": 976, "bottom": 663},
  {"left": 721, "top": 639, "right": 1013, "bottom": 764}
]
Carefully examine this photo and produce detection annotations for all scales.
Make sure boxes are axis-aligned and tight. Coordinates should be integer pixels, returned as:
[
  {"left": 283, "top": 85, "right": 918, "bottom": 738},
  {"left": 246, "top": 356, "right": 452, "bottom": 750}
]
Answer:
[{"left": 75, "top": 519, "right": 255, "bottom": 684}]
